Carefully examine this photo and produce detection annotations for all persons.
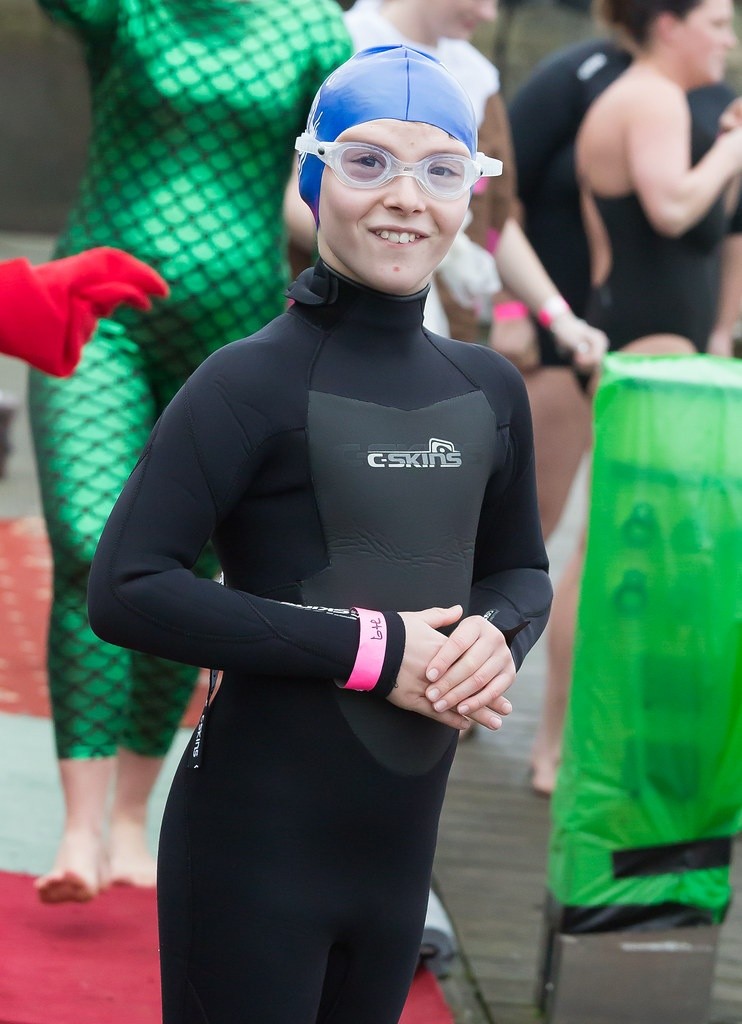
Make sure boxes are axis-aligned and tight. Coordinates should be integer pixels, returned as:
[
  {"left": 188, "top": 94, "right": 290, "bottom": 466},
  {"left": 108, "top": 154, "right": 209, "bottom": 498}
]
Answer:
[
  {"left": 0, "top": 0, "right": 742, "bottom": 1024},
  {"left": 89, "top": 47, "right": 553, "bottom": 1024}
]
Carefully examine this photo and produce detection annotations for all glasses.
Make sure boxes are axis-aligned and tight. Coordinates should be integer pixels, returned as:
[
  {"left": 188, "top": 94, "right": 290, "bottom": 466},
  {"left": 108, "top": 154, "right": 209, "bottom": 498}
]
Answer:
[{"left": 297, "top": 131, "right": 505, "bottom": 203}]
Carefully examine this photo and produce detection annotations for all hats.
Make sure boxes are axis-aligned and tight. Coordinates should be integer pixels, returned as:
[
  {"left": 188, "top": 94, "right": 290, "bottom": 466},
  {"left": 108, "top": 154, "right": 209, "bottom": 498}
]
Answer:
[{"left": 297, "top": 44, "right": 484, "bottom": 232}]
